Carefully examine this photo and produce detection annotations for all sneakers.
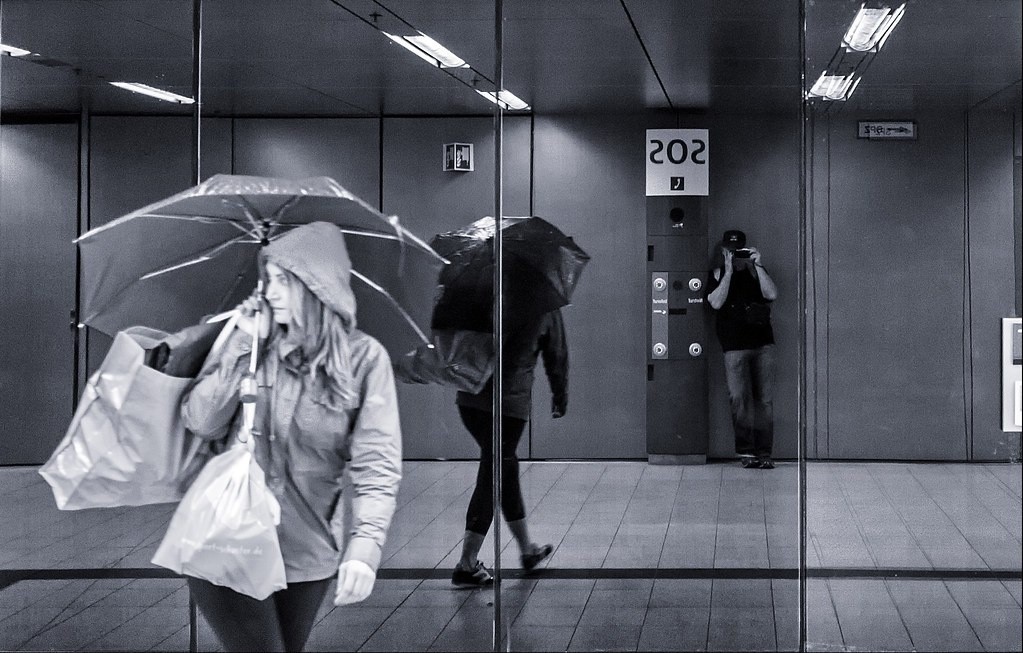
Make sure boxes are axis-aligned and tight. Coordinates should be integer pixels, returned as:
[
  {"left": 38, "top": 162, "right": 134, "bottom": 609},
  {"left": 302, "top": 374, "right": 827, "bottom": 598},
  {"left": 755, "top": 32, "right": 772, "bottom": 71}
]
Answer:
[
  {"left": 758, "top": 457, "right": 775, "bottom": 468},
  {"left": 450, "top": 560, "right": 493, "bottom": 589},
  {"left": 521, "top": 543, "right": 553, "bottom": 569},
  {"left": 741, "top": 457, "right": 759, "bottom": 467}
]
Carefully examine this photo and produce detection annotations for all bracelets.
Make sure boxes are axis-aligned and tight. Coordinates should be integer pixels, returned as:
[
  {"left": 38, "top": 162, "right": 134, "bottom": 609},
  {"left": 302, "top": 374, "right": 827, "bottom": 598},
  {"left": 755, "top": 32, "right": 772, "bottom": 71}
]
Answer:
[{"left": 754, "top": 262, "right": 763, "bottom": 268}]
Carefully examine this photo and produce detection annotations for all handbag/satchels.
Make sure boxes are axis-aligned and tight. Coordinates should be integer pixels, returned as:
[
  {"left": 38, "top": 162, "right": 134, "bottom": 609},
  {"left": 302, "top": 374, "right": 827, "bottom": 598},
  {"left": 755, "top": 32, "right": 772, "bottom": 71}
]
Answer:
[
  {"left": 36, "top": 307, "right": 244, "bottom": 509},
  {"left": 395, "top": 329, "right": 494, "bottom": 395},
  {"left": 151, "top": 435, "right": 287, "bottom": 600}
]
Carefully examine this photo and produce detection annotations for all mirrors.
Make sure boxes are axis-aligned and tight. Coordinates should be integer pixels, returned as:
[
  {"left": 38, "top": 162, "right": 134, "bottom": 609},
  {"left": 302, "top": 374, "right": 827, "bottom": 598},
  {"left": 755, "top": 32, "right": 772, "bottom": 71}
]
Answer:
[{"left": 0, "top": 1, "right": 1023, "bottom": 653}]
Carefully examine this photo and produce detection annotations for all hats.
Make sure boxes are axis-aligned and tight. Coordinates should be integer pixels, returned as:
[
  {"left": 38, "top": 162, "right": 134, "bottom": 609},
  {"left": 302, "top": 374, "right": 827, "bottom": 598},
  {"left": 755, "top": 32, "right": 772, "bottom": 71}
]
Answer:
[{"left": 722, "top": 230, "right": 746, "bottom": 250}]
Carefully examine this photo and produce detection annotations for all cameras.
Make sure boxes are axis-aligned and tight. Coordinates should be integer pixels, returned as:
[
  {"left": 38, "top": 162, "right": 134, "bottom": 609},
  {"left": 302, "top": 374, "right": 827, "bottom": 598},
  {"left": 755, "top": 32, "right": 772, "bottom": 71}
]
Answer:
[{"left": 729, "top": 248, "right": 753, "bottom": 259}]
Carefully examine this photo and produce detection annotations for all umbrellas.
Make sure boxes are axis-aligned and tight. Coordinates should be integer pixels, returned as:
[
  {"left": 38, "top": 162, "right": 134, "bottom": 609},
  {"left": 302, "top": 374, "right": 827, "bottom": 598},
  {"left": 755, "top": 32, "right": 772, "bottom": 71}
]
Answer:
[
  {"left": 426, "top": 216, "right": 591, "bottom": 333},
  {"left": 71, "top": 174, "right": 451, "bottom": 404}
]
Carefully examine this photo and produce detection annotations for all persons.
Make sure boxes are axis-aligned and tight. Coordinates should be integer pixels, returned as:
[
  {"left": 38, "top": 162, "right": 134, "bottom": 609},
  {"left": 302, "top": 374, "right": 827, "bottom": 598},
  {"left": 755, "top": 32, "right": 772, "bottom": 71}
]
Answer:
[
  {"left": 450, "top": 309, "right": 569, "bottom": 589},
  {"left": 180, "top": 222, "right": 403, "bottom": 653},
  {"left": 705, "top": 231, "right": 778, "bottom": 469}
]
